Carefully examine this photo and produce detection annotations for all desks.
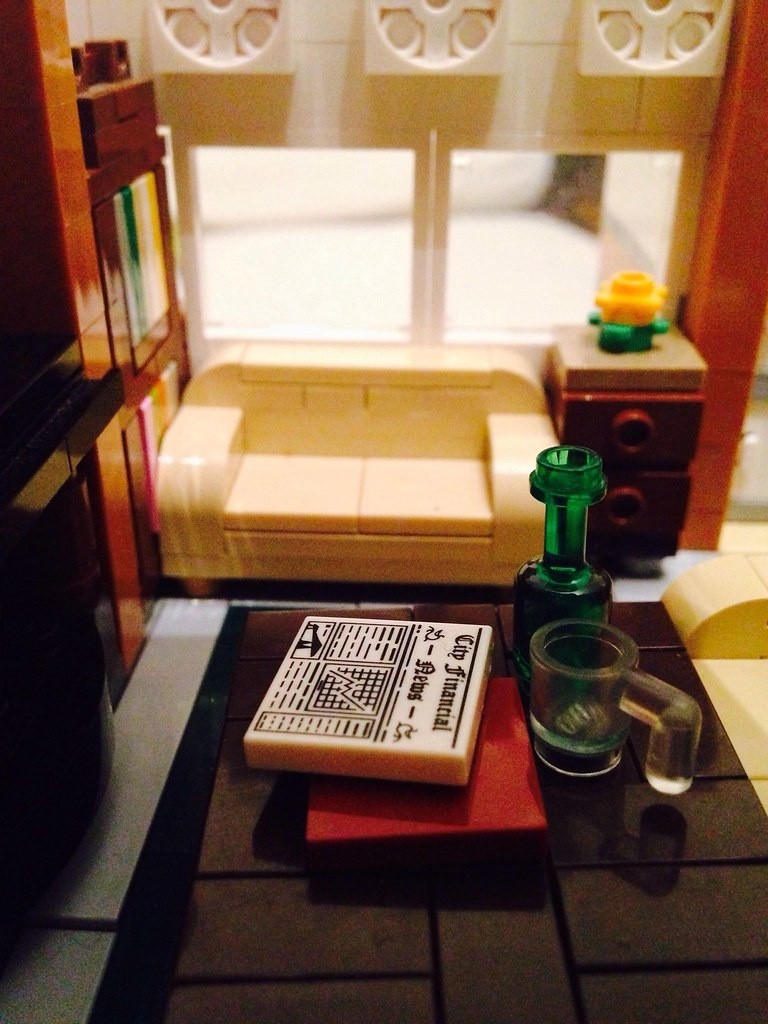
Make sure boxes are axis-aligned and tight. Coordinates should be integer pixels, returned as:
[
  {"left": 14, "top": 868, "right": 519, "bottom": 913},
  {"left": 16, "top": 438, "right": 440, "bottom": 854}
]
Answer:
[{"left": 0, "top": 600, "right": 768, "bottom": 1024}]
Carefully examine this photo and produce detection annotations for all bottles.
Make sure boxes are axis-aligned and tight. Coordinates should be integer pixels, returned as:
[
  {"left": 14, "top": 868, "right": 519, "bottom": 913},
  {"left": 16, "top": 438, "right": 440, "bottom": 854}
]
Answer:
[{"left": 513, "top": 444, "right": 614, "bottom": 702}]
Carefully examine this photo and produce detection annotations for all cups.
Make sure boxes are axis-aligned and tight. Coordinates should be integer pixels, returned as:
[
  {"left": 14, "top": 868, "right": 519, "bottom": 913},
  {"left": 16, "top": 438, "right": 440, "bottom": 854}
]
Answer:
[{"left": 531, "top": 618, "right": 701, "bottom": 794}]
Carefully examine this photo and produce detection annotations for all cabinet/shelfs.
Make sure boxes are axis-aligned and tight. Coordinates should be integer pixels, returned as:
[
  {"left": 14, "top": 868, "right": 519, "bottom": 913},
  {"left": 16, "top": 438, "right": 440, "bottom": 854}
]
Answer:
[
  {"left": 548, "top": 321, "right": 707, "bottom": 562},
  {"left": 84, "top": 159, "right": 190, "bottom": 597},
  {"left": 0, "top": 0, "right": 147, "bottom": 673}
]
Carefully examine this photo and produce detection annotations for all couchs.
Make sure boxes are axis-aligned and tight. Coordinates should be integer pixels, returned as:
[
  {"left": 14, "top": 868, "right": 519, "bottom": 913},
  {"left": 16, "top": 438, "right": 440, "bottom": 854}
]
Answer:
[{"left": 150, "top": 341, "right": 559, "bottom": 600}]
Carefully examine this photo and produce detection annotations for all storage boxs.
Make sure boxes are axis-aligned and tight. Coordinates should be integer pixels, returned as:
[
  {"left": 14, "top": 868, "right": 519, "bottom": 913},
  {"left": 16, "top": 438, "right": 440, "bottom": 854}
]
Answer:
[{"left": 73, "top": 78, "right": 166, "bottom": 166}]
